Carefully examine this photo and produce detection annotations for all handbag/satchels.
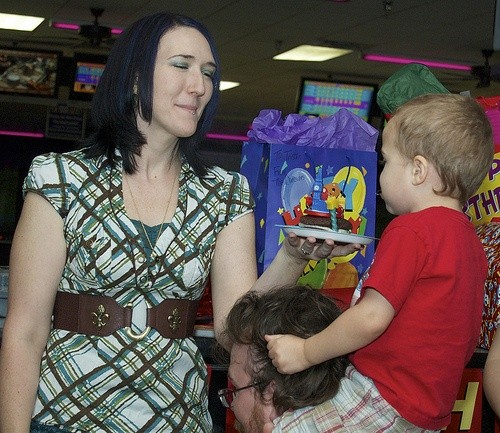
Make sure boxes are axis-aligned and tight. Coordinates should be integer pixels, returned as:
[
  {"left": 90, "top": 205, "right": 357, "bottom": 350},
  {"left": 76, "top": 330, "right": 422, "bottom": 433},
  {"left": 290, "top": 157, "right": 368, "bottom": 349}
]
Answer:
[{"left": 239, "top": 139, "right": 378, "bottom": 289}]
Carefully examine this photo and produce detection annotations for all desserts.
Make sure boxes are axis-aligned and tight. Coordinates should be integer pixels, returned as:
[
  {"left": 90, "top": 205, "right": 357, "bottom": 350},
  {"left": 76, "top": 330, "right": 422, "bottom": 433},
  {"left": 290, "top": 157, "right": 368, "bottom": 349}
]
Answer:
[{"left": 298, "top": 166, "right": 353, "bottom": 234}]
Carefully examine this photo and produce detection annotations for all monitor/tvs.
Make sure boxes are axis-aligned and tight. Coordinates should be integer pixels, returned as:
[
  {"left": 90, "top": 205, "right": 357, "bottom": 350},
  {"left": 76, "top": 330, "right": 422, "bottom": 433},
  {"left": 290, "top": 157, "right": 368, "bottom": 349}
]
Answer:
[
  {"left": 0, "top": 45, "right": 62, "bottom": 98},
  {"left": 295, "top": 78, "right": 380, "bottom": 126},
  {"left": 68, "top": 52, "right": 110, "bottom": 101}
]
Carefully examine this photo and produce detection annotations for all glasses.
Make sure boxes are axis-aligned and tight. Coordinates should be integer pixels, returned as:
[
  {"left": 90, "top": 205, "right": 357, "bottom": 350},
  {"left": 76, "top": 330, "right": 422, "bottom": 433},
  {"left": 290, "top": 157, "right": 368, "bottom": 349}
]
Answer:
[{"left": 218, "top": 380, "right": 270, "bottom": 409}]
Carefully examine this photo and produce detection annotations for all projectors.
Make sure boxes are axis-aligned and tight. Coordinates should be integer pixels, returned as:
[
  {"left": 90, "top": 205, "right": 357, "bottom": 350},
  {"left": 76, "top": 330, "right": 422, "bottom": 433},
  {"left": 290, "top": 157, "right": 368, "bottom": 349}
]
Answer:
[{"left": 79, "top": 25, "right": 112, "bottom": 38}]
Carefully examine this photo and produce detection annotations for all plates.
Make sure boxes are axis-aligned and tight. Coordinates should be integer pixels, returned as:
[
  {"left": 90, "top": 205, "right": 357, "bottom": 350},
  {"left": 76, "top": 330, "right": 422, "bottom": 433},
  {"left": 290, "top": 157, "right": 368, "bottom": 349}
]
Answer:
[{"left": 274, "top": 225, "right": 381, "bottom": 245}]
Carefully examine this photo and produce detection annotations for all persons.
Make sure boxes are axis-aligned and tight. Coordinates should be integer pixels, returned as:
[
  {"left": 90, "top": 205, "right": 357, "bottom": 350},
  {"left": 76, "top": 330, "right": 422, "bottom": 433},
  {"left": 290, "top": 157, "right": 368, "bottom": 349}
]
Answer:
[
  {"left": 212, "top": 284, "right": 351, "bottom": 433},
  {"left": 0, "top": 11, "right": 364, "bottom": 433},
  {"left": 265, "top": 93, "right": 495, "bottom": 433}
]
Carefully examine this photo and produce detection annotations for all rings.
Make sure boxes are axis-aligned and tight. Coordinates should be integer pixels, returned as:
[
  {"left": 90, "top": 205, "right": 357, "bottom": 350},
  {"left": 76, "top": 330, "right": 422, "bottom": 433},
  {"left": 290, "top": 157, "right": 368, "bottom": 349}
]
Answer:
[{"left": 301, "top": 249, "right": 312, "bottom": 255}]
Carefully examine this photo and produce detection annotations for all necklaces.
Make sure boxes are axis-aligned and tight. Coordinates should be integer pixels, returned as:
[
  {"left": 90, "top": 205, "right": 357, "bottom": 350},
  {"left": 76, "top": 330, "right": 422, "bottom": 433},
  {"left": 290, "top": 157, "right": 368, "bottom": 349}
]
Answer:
[{"left": 123, "top": 170, "right": 178, "bottom": 252}]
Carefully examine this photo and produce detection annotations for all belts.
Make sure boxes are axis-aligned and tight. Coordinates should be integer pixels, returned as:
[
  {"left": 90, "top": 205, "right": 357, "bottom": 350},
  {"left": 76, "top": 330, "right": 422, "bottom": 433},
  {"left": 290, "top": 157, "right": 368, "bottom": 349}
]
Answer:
[{"left": 52, "top": 292, "right": 196, "bottom": 340}]
